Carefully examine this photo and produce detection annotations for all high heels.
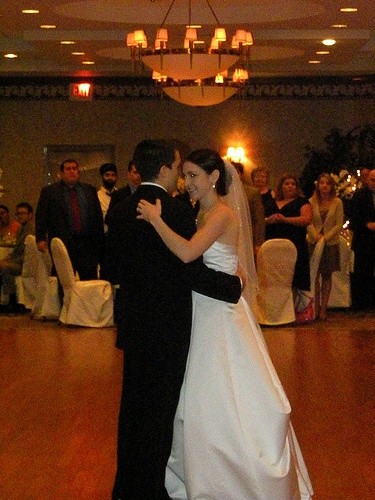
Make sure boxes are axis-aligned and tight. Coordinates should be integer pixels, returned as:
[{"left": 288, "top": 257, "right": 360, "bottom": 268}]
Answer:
[{"left": 312, "top": 306, "right": 328, "bottom": 321}]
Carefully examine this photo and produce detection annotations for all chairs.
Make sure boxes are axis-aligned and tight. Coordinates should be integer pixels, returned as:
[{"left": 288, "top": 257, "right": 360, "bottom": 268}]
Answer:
[
  {"left": 15, "top": 234, "right": 114, "bottom": 328},
  {"left": 254, "top": 235, "right": 354, "bottom": 325}
]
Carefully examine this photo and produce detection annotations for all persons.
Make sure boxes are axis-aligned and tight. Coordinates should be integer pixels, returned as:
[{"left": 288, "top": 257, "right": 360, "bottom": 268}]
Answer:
[
  {"left": 107, "top": 139, "right": 246, "bottom": 500},
  {"left": 136, "top": 148, "right": 260, "bottom": 500},
  {"left": 0, "top": 159, "right": 375, "bottom": 321}
]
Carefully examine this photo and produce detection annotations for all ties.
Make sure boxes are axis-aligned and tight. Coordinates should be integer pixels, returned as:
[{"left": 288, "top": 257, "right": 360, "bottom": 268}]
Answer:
[{"left": 70, "top": 189, "right": 80, "bottom": 234}]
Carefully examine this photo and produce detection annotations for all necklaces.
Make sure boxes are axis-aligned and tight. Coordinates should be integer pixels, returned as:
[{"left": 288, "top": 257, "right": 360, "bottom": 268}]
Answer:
[{"left": 195, "top": 201, "right": 219, "bottom": 225}]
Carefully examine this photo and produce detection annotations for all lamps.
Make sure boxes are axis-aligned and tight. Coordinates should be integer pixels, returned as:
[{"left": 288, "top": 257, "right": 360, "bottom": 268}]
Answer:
[{"left": 126, "top": 0, "right": 254, "bottom": 106}]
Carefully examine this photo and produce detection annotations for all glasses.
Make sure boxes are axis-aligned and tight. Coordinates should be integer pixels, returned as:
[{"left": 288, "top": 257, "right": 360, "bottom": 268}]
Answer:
[{"left": 14, "top": 211, "right": 30, "bottom": 216}]
